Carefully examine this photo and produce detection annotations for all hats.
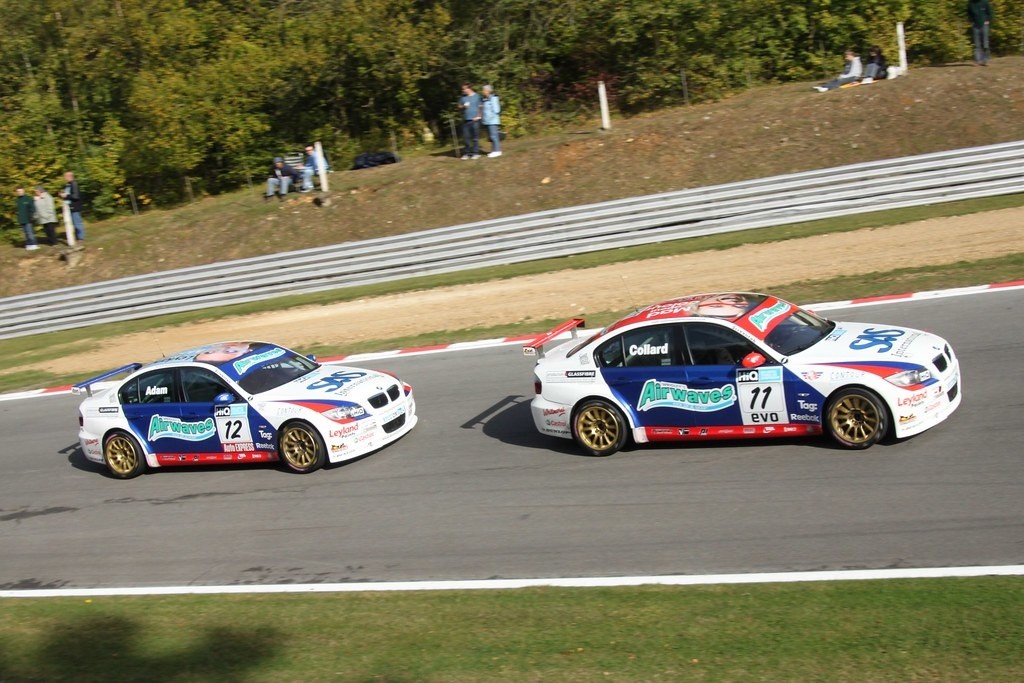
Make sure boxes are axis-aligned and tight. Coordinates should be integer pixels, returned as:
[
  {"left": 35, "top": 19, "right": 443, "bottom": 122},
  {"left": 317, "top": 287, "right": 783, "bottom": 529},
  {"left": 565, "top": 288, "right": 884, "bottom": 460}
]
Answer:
[{"left": 272, "top": 157, "right": 283, "bottom": 163}]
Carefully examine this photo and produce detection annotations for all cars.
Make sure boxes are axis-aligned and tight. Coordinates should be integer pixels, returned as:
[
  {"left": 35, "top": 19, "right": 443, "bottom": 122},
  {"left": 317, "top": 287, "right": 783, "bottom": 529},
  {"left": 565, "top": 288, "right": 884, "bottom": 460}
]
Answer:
[
  {"left": 72, "top": 340, "right": 418, "bottom": 479},
  {"left": 522, "top": 291, "right": 962, "bottom": 456}
]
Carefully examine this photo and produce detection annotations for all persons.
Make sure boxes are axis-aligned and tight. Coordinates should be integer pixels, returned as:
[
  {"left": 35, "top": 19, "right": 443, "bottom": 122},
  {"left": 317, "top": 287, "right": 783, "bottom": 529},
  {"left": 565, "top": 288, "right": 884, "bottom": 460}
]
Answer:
[
  {"left": 13, "top": 171, "right": 85, "bottom": 250},
  {"left": 812, "top": 45, "right": 888, "bottom": 92},
  {"left": 458, "top": 82, "right": 502, "bottom": 160},
  {"left": 266, "top": 144, "right": 330, "bottom": 202},
  {"left": 967, "top": 0, "right": 992, "bottom": 67}
]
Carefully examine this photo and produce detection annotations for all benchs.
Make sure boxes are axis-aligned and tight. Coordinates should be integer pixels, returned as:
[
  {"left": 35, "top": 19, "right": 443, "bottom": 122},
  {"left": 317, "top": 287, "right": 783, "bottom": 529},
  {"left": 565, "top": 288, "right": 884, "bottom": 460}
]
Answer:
[{"left": 282, "top": 157, "right": 333, "bottom": 186}]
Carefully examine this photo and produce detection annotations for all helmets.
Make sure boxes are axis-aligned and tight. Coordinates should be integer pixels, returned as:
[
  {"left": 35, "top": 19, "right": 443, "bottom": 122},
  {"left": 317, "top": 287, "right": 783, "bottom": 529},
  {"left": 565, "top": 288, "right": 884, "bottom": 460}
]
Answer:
[{"left": 768, "top": 325, "right": 794, "bottom": 347}]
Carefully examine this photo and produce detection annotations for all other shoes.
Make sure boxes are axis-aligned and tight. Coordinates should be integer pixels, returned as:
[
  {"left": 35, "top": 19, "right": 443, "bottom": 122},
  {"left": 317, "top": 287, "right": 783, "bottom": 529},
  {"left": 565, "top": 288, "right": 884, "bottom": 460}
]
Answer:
[
  {"left": 861, "top": 77, "right": 873, "bottom": 85},
  {"left": 981, "top": 56, "right": 992, "bottom": 65},
  {"left": 47, "top": 242, "right": 58, "bottom": 247},
  {"left": 76, "top": 240, "right": 84, "bottom": 244},
  {"left": 973, "top": 61, "right": 983, "bottom": 66},
  {"left": 25, "top": 244, "right": 41, "bottom": 251},
  {"left": 470, "top": 154, "right": 481, "bottom": 160},
  {"left": 298, "top": 188, "right": 311, "bottom": 193},
  {"left": 280, "top": 194, "right": 287, "bottom": 202},
  {"left": 460, "top": 154, "right": 469, "bottom": 161},
  {"left": 265, "top": 196, "right": 273, "bottom": 202},
  {"left": 487, "top": 151, "right": 502, "bottom": 158},
  {"left": 817, "top": 87, "right": 829, "bottom": 92},
  {"left": 812, "top": 86, "right": 821, "bottom": 89}
]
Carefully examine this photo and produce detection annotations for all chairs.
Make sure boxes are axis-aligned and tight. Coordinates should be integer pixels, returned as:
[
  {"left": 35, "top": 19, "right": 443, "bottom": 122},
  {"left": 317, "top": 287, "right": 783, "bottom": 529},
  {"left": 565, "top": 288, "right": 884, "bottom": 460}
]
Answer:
[{"left": 717, "top": 347, "right": 738, "bottom": 366}]
